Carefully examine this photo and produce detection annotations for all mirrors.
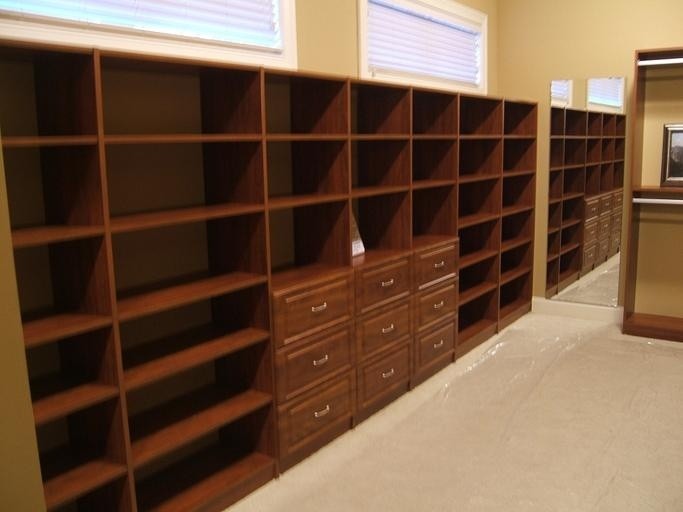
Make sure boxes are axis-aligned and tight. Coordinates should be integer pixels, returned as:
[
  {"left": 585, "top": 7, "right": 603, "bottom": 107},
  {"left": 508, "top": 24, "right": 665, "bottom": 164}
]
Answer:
[{"left": 544, "top": 76, "right": 627, "bottom": 307}]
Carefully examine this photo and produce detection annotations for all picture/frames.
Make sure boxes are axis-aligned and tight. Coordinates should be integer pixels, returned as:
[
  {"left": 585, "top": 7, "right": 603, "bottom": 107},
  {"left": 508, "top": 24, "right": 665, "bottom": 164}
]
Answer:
[{"left": 660, "top": 124, "right": 683, "bottom": 187}]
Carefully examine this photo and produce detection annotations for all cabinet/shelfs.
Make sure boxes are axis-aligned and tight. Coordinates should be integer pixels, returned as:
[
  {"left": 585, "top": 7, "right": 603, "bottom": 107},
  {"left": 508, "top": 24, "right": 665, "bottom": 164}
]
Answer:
[
  {"left": 1, "top": 36, "right": 276, "bottom": 511},
  {"left": 546, "top": 107, "right": 626, "bottom": 299},
  {"left": 460, "top": 93, "right": 538, "bottom": 356},
  {"left": 622, "top": 48, "right": 683, "bottom": 343},
  {"left": 263, "top": 68, "right": 459, "bottom": 474}
]
